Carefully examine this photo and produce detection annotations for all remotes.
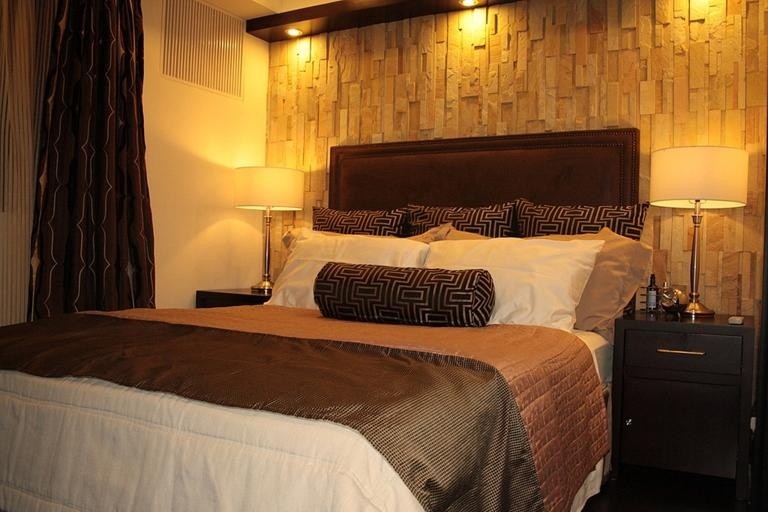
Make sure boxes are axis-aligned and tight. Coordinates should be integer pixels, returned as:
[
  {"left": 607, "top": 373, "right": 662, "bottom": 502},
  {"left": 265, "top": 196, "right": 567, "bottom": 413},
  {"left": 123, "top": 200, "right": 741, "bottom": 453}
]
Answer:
[{"left": 729, "top": 316, "right": 744, "bottom": 324}]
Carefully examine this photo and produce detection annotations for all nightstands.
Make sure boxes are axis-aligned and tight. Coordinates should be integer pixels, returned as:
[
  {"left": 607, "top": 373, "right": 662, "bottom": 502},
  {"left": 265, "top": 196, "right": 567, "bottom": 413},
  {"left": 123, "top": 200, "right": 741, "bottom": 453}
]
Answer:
[
  {"left": 196, "top": 289, "right": 272, "bottom": 309},
  {"left": 612, "top": 305, "right": 755, "bottom": 502}
]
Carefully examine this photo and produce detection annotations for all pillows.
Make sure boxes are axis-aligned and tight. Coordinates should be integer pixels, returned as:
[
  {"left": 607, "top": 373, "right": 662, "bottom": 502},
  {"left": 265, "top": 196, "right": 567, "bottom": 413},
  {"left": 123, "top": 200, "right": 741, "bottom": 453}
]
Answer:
[
  {"left": 308, "top": 203, "right": 409, "bottom": 242},
  {"left": 436, "top": 227, "right": 651, "bottom": 327},
  {"left": 408, "top": 201, "right": 518, "bottom": 243},
  {"left": 312, "top": 260, "right": 498, "bottom": 329},
  {"left": 425, "top": 235, "right": 605, "bottom": 328},
  {"left": 513, "top": 196, "right": 652, "bottom": 243},
  {"left": 264, "top": 228, "right": 431, "bottom": 316},
  {"left": 281, "top": 219, "right": 452, "bottom": 266}
]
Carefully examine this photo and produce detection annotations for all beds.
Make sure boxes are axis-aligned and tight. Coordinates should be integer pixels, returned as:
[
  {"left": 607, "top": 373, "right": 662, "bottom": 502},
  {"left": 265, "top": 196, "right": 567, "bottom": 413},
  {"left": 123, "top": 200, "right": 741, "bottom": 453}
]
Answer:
[{"left": 1, "top": 124, "right": 639, "bottom": 512}]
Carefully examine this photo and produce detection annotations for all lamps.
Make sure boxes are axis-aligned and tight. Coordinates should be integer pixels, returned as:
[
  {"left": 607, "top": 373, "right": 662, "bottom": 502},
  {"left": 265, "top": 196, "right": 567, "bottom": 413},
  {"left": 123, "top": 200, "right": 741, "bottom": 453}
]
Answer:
[
  {"left": 235, "top": 165, "right": 305, "bottom": 293},
  {"left": 649, "top": 147, "right": 749, "bottom": 318}
]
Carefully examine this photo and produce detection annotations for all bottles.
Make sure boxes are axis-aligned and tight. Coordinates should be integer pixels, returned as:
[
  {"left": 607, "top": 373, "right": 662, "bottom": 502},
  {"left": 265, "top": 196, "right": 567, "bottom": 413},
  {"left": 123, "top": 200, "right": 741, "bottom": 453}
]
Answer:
[{"left": 646, "top": 274, "right": 669, "bottom": 313}]
